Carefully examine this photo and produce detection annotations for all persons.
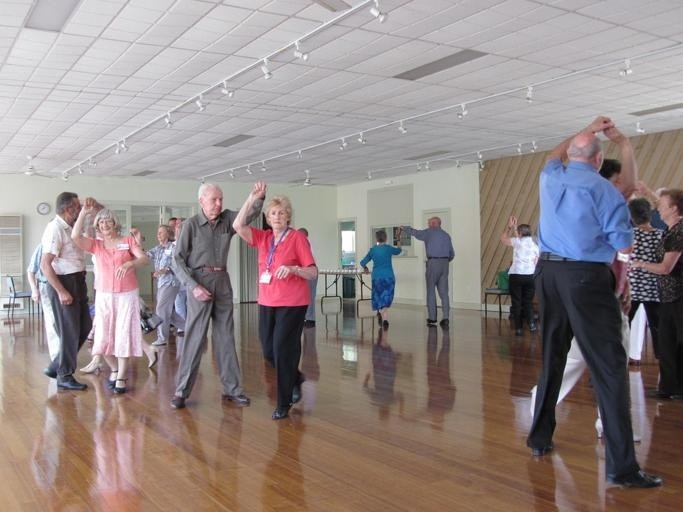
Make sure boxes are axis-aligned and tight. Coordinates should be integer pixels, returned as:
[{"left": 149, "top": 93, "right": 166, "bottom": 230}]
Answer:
[
  {"left": 400, "top": 217, "right": 455, "bottom": 326},
  {"left": 29, "top": 345, "right": 181, "bottom": 512},
  {"left": 263, "top": 420, "right": 308, "bottom": 512},
  {"left": 171, "top": 181, "right": 268, "bottom": 410},
  {"left": 292, "top": 328, "right": 321, "bottom": 416},
  {"left": 360, "top": 230, "right": 401, "bottom": 330},
  {"left": 297, "top": 229, "right": 318, "bottom": 328},
  {"left": 27, "top": 191, "right": 188, "bottom": 395},
  {"left": 177, "top": 411, "right": 240, "bottom": 512},
  {"left": 526, "top": 438, "right": 662, "bottom": 512},
  {"left": 362, "top": 331, "right": 406, "bottom": 423},
  {"left": 501, "top": 215, "right": 541, "bottom": 336},
  {"left": 234, "top": 183, "right": 317, "bottom": 420},
  {"left": 427, "top": 326, "right": 456, "bottom": 430},
  {"left": 527, "top": 116, "right": 682, "bottom": 487}
]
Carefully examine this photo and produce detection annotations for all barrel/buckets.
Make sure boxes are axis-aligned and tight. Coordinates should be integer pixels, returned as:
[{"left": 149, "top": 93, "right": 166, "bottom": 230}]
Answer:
[{"left": 495, "top": 271, "right": 510, "bottom": 290}]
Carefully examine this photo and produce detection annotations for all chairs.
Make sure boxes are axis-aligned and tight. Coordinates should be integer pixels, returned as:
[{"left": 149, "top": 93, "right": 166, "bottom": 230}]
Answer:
[{"left": 5, "top": 274, "right": 35, "bottom": 318}]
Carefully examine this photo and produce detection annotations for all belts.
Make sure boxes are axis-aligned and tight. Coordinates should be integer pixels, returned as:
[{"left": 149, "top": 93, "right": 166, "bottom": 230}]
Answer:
[
  {"left": 541, "top": 254, "right": 572, "bottom": 262},
  {"left": 204, "top": 267, "right": 224, "bottom": 272}
]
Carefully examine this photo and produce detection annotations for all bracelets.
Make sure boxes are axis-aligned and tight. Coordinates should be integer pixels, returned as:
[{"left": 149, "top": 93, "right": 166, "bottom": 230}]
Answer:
[
  {"left": 364, "top": 266, "right": 367, "bottom": 269},
  {"left": 397, "top": 239, "right": 400, "bottom": 242}
]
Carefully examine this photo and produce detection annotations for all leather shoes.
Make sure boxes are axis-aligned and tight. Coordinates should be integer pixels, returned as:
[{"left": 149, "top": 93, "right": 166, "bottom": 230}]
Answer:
[
  {"left": 45, "top": 369, "right": 87, "bottom": 390},
  {"left": 152, "top": 339, "right": 167, "bottom": 345},
  {"left": 107, "top": 370, "right": 127, "bottom": 393},
  {"left": 426, "top": 319, "right": 449, "bottom": 325},
  {"left": 532, "top": 441, "right": 553, "bottom": 456},
  {"left": 169, "top": 397, "right": 185, "bottom": 409},
  {"left": 606, "top": 469, "right": 662, "bottom": 487},
  {"left": 377, "top": 312, "right": 389, "bottom": 328},
  {"left": 271, "top": 375, "right": 304, "bottom": 418},
  {"left": 221, "top": 394, "right": 250, "bottom": 404}
]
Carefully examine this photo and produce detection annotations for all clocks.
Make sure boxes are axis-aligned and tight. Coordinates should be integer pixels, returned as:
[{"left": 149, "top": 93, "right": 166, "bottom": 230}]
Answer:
[{"left": 34, "top": 201, "right": 51, "bottom": 216}]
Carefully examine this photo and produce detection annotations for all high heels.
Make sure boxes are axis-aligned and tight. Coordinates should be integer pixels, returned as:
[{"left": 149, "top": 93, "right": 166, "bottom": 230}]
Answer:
[
  {"left": 146, "top": 345, "right": 160, "bottom": 368},
  {"left": 79, "top": 360, "right": 103, "bottom": 373}
]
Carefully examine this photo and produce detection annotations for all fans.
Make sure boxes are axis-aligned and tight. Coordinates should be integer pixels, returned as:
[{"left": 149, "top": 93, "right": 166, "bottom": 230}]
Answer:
[{"left": 0, "top": 155, "right": 57, "bottom": 181}]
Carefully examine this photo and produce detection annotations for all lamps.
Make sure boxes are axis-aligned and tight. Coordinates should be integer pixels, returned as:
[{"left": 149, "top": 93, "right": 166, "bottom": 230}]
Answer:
[
  {"left": 229, "top": 161, "right": 266, "bottom": 179},
  {"left": 339, "top": 137, "right": 349, "bottom": 151},
  {"left": 197, "top": 99, "right": 206, "bottom": 111},
  {"left": 260, "top": 66, "right": 272, "bottom": 81},
  {"left": 457, "top": 105, "right": 468, "bottom": 120},
  {"left": 416, "top": 153, "right": 485, "bottom": 182},
  {"left": 635, "top": 121, "right": 644, "bottom": 133},
  {"left": 221, "top": 88, "right": 235, "bottom": 98},
  {"left": 516, "top": 140, "right": 537, "bottom": 155},
  {"left": 619, "top": 59, "right": 633, "bottom": 77},
  {"left": 165, "top": 117, "right": 173, "bottom": 130},
  {"left": 115, "top": 139, "right": 128, "bottom": 155},
  {"left": 358, "top": 132, "right": 366, "bottom": 145},
  {"left": 303, "top": 171, "right": 312, "bottom": 186},
  {"left": 398, "top": 122, "right": 407, "bottom": 134},
  {"left": 293, "top": 51, "right": 308, "bottom": 62},
  {"left": 297, "top": 149, "right": 303, "bottom": 160},
  {"left": 366, "top": 171, "right": 373, "bottom": 181},
  {"left": 200, "top": 178, "right": 205, "bottom": 186},
  {"left": 62, "top": 159, "right": 96, "bottom": 182},
  {"left": 525, "top": 85, "right": 533, "bottom": 103},
  {"left": 369, "top": 8, "right": 385, "bottom": 23}
]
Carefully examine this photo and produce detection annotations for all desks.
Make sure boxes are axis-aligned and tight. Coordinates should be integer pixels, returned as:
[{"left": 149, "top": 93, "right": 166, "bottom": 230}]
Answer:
[
  {"left": 316, "top": 268, "right": 373, "bottom": 306},
  {"left": 483, "top": 287, "right": 511, "bottom": 318}
]
